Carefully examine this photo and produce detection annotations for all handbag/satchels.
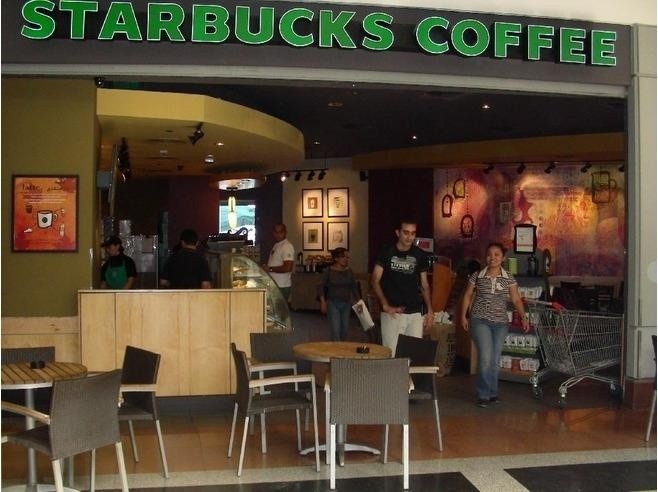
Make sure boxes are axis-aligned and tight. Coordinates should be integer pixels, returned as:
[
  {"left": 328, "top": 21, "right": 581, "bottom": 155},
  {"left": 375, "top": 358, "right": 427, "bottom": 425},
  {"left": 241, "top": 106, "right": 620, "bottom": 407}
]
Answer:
[{"left": 316, "top": 266, "right": 331, "bottom": 303}]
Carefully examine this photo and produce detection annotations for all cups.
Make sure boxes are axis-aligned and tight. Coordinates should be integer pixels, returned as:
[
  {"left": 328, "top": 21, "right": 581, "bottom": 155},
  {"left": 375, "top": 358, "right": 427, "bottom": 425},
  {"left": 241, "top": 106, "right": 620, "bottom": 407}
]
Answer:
[{"left": 38, "top": 211, "right": 58, "bottom": 228}]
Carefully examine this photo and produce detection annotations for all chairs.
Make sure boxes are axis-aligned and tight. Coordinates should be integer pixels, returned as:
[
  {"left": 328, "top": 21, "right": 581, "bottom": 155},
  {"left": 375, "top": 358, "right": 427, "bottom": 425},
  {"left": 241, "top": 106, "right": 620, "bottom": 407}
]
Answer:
[
  {"left": 1, "top": 367, "right": 130, "bottom": 492},
  {"left": 395, "top": 333, "right": 443, "bottom": 452},
  {"left": 249, "top": 332, "right": 317, "bottom": 431},
  {"left": 90, "top": 345, "right": 170, "bottom": 492},
  {"left": 324, "top": 357, "right": 414, "bottom": 489},
  {"left": 227, "top": 343, "right": 320, "bottom": 478}
]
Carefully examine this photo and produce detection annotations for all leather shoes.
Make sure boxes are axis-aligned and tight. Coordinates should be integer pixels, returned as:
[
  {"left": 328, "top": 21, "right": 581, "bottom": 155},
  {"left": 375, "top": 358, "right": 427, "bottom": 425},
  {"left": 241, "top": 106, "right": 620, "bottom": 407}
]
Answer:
[
  {"left": 478, "top": 399, "right": 488, "bottom": 407},
  {"left": 490, "top": 396, "right": 501, "bottom": 404}
]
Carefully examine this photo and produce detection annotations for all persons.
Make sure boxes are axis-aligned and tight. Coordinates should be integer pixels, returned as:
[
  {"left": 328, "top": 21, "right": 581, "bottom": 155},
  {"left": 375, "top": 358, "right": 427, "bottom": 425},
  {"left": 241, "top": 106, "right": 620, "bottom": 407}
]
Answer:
[
  {"left": 159, "top": 229, "right": 212, "bottom": 289},
  {"left": 100, "top": 236, "right": 136, "bottom": 289},
  {"left": 461, "top": 242, "right": 530, "bottom": 407},
  {"left": 263, "top": 223, "right": 295, "bottom": 300},
  {"left": 372, "top": 220, "right": 435, "bottom": 401},
  {"left": 317, "top": 248, "right": 361, "bottom": 342}
]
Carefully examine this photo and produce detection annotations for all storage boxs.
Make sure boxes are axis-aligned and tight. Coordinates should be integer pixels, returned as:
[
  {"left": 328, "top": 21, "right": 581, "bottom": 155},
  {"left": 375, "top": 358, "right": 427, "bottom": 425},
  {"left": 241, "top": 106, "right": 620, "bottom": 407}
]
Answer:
[{"left": 427, "top": 323, "right": 467, "bottom": 377}]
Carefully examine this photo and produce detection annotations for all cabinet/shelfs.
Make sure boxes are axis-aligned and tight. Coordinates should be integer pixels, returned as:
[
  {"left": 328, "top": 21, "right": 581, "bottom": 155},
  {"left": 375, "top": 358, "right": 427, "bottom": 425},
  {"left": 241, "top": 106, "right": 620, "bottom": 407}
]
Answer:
[{"left": 206, "top": 249, "right": 290, "bottom": 332}]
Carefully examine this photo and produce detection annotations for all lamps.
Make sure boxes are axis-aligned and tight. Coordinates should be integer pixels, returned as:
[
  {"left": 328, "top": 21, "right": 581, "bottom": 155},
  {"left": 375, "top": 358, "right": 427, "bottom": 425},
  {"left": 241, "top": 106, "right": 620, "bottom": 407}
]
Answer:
[
  {"left": 294, "top": 171, "right": 301, "bottom": 181},
  {"left": 226, "top": 187, "right": 238, "bottom": 230},
  {"left": 307, "top": 171, "right": 315, "bottom": 180},
  {"left": 317, "top": 170, "right": 326, "bottom": 180},
  {"left": 280, "top": 171, "right": 287, "bottom": 182},
  {"left": 187, "top": 122, "right": 204, "bottom": 145}
]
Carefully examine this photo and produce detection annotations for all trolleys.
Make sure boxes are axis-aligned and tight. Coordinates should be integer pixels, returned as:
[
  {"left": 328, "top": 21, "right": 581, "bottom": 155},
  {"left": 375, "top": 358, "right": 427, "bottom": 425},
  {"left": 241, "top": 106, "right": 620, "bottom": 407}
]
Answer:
[{"left": 519, "top": 296, "right": 626, "bottom": 409}]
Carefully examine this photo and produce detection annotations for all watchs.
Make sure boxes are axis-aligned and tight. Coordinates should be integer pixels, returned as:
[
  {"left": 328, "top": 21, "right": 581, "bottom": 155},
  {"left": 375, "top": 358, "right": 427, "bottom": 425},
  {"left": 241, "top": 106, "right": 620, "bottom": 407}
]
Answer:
[{"left": 269, "top": 266, "right": 273, "bottom": 272}]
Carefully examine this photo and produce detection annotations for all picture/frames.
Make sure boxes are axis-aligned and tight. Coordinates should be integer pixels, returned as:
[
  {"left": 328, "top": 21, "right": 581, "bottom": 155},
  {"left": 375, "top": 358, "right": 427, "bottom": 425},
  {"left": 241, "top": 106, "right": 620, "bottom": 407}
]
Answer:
[
  {"left": 327, "top": 222, "right": 350, "bottom": 251},
  {"left": 302, "top": 188, "right": 323, "bottom": 218},
  {"left": 10, "top": 175, "right": 80, "bottom": 253},
  {"left": 302, "top": 221, "right": 323, "bottom": 250},
  {"left": 327, "top": 187, "right": 350, "bottom": 218},
  {"left": 512, "top": 223, "right": 537, "bottom": 254}
]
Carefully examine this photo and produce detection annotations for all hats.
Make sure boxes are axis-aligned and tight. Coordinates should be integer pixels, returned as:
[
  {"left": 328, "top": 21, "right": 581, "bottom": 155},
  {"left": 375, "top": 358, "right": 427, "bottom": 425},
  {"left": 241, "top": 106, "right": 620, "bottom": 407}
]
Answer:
[{"left": 100, "top": 236, "right": 122, "bottom": 247}]
